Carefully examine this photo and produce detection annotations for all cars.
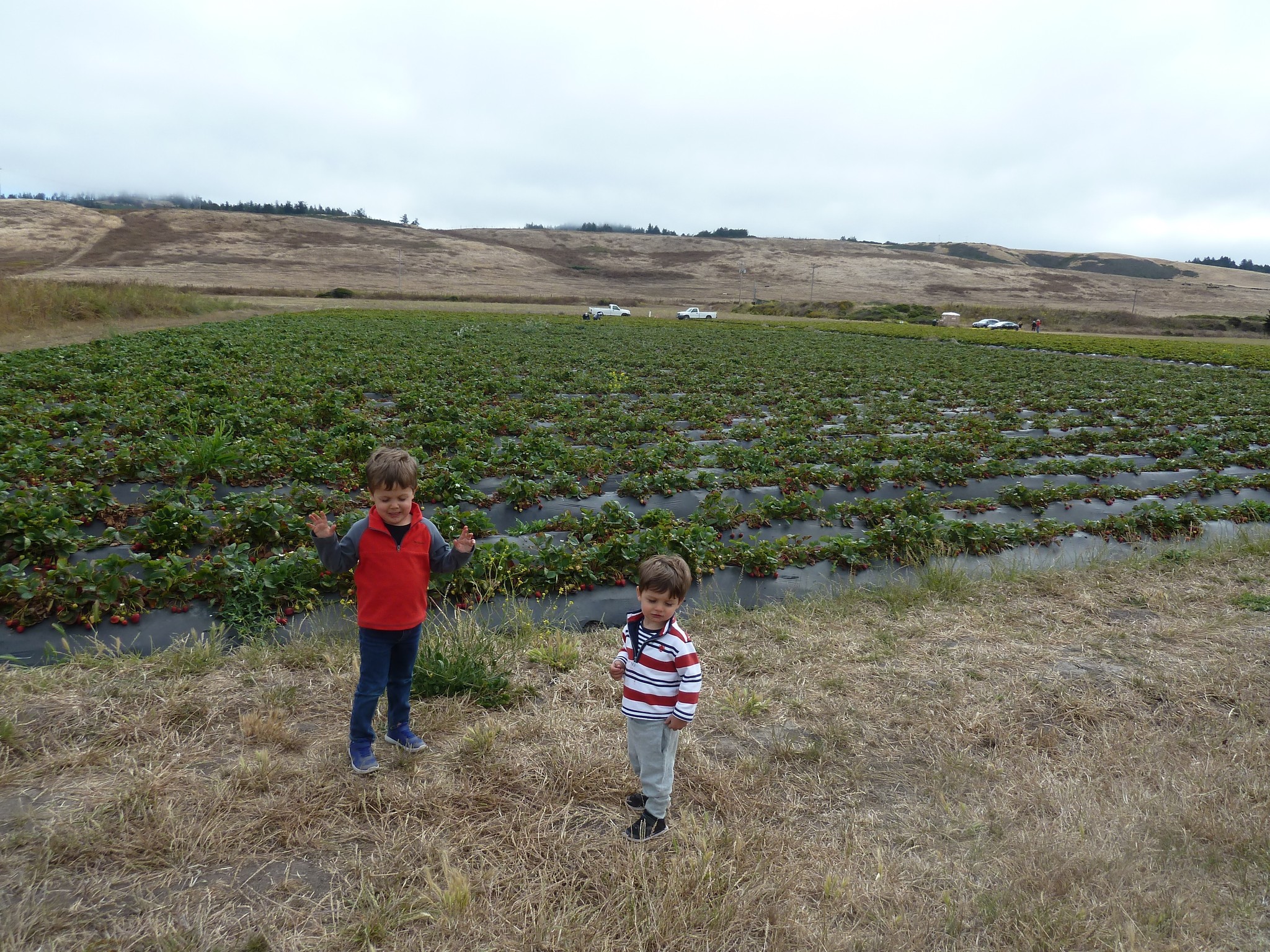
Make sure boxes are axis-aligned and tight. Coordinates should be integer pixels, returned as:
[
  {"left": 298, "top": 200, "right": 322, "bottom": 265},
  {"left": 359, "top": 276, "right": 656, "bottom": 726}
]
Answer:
[
  {"left": 972, "top": 319, "right": 1000, "bottom": 328},
  {"left": 987, "top": 321, "right": 1020, "bottom": 332}
]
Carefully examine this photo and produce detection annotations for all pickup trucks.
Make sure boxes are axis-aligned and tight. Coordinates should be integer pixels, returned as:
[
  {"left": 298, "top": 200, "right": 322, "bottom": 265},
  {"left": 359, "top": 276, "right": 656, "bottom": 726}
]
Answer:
[
  {"left": 588, "top": 303, "right": 631, "bottom": 317},
  {"left": 675, "top": 307, "right": 717, "bottom": 321}
]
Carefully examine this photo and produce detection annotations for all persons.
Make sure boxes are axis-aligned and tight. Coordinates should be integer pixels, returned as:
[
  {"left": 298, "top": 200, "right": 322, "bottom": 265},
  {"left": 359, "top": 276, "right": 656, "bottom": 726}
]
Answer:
[
  {"left": 306, "top": 446, "right": 476, "bottom": 774},
  {"left": 1018, "top": 319, "right": 1041, "bottom": 332},
  {"left": 583, "top": 313, "right": 590, "bottom": 320},
  {"left": 609, "top": 554, "right": 702, "bottom": 842},
  {"left": 593, "top": 313, "right": 603, "bottom": 320}
]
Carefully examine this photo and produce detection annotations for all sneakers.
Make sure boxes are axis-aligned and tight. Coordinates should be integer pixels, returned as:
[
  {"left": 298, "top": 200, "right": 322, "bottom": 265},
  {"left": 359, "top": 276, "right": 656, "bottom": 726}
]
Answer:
[
  {"left": 384, "top": 722, "right": 427, "bottom": 752},
  {"left": 348, "top": 740, "right": 379, "bottom": 774},
  {"left": 625, "top": 792, "right": 649, "bottom": 810},
  {"left": 622, "top": 809, "right": 668, "bottom": 842}
]
